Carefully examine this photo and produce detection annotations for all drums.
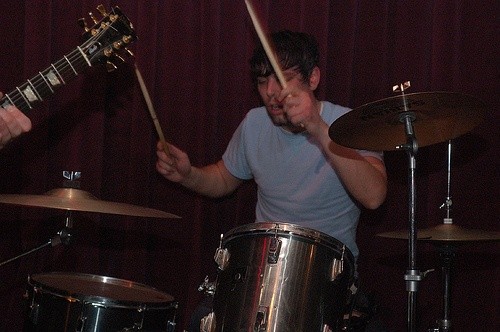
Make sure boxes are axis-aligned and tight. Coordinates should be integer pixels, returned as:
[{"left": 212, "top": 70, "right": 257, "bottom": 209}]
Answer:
[
  {"left": 198, "top": 221, "right": 361, "bottom": 332},
  {"left": 23, "top": 270, "right": 184, "bottom": 332}
]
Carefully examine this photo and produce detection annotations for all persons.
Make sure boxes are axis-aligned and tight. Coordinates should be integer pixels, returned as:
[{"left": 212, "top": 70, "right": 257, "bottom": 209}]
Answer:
[
  {"left": 156, "top": 28, "right": 386, "bottom": 325},
  {"left": 0, "top": 90, "right": 31, "bottom": 151}
]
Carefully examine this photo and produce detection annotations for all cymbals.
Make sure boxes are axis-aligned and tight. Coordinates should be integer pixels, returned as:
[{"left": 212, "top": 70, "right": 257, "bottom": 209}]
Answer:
[
  {"left": 328, "top": 91, "right": 491, "bottom": 151},
  {"left": 1, "top": 188, "right": 181, "bottom": 218},
  {"left": 376, "top": 225, "right": 500, "bottom": 241}
]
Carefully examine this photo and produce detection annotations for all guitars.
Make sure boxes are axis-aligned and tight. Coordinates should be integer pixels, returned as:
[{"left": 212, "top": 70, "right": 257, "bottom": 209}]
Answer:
[{"left": 0, "top": 4, "right": 139, "bottom": 114}]
